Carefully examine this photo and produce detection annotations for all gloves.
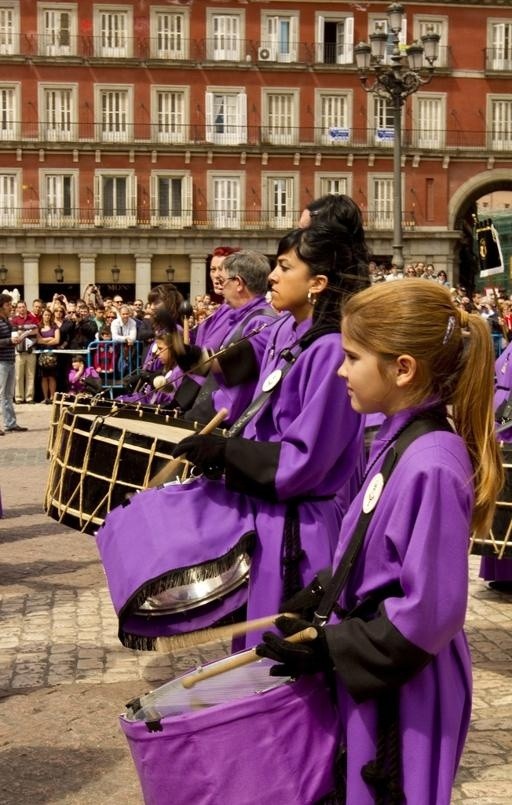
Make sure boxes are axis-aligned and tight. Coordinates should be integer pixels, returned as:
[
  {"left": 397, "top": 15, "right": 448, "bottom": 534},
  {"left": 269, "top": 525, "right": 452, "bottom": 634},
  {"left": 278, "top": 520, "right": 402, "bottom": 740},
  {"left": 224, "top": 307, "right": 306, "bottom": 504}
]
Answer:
[
  {"left": 256, "top": 616, "right": 323, "bottom": 674},
  {"left": 277, "top": 571, "right": 330, "bottom": 625},
  {"left": 174, "top": 436, "right": 228, "bottom": 479}
]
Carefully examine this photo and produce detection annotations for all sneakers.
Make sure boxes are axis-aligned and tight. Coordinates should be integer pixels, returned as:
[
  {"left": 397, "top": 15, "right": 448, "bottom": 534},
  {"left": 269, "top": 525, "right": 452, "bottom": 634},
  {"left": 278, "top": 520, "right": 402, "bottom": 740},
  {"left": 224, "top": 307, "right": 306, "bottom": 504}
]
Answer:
[{"left": 5, "top": 425, "right": 27, "bottom": 433}]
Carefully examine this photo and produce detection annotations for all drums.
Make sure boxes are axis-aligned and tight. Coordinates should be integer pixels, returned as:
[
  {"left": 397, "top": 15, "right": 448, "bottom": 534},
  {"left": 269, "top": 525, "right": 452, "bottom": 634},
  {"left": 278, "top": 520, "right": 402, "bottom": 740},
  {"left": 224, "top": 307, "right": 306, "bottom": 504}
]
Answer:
[
  {"left": 125, "top": 643, "right": 341, "bottom": 805},
  {"left": 463, "top": 441, "right": 511, "bottom": 558},
  {"left": 43, "top": 392, "right": 241, "bottom": 537},
  {"left": 100, "top": 481, "right": 258, "bottom": 617}
]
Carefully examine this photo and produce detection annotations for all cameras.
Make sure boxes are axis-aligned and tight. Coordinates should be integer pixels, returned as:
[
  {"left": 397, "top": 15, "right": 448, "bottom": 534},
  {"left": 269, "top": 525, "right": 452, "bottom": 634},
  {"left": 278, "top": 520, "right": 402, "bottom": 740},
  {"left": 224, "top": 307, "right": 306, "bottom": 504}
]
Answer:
[
  {"left": 92, "top": 288, "right": 98, "bottom": 294},
  {"left": 57, "top": 294, "right": 63, "bottom": 299}
]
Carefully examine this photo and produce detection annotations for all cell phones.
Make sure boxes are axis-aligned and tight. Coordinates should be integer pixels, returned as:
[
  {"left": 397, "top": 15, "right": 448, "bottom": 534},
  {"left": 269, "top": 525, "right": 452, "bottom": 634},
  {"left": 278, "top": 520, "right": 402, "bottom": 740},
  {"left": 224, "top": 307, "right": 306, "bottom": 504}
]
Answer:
[{"left": 88, "top": 283, "right": 93, "bottom": 287}]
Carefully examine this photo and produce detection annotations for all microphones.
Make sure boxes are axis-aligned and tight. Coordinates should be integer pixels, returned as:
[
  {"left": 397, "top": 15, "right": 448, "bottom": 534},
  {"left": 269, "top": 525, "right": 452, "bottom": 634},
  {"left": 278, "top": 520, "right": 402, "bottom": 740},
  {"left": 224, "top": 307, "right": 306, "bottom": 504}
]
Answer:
[{"left": 153, "top": 375, "right": 178, "bottom": 395}]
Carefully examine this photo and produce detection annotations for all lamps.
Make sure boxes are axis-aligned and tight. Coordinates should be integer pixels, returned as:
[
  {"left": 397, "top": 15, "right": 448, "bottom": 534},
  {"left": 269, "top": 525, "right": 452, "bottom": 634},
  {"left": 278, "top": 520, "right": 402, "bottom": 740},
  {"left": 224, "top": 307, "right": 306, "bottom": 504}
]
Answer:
[
  {"left": 166, "top": 254, "right": 175, "bottom": 281},
  {"left": 0, "top": 254, "right": 9, "bottom": 285},
  {"left": 55, "top": 254, "right": 64, "bottom": 282},
  {"left": 111, "top": 254, "right": 120, "bottom": 282}
]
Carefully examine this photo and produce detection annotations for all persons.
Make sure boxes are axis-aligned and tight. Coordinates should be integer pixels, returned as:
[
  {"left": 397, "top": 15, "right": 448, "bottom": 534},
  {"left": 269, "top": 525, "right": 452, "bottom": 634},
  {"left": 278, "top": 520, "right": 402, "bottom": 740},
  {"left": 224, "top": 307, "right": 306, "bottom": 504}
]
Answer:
[
  {"left": 107, "top": 196, "right": 512, "bottom": 805},
  {"left": 0, "top": 282, "right": 214, "bottom": 435}
]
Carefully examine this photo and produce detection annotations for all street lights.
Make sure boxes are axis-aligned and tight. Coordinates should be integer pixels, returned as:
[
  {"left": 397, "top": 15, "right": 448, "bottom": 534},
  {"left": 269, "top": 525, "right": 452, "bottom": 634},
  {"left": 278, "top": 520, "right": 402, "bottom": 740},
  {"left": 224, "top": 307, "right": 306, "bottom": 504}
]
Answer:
[{"left": 353, "top": 4, "right": 439, "bottom": 271}]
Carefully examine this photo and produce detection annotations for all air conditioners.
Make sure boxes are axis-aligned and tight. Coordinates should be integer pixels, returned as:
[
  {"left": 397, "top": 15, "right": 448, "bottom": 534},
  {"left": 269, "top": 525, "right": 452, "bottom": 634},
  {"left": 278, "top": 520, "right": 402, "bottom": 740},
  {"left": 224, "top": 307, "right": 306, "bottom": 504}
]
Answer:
[{"left": 258, "top": 47, "right": 276, "bottom": 63}]
